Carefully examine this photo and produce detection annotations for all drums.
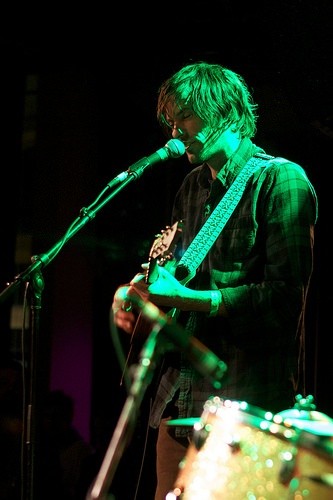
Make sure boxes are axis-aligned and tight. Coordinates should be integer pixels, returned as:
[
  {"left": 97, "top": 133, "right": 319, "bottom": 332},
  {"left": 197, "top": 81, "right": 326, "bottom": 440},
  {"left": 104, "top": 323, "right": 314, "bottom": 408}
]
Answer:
[{"left": 165, "top": 393, "right": 333, "bottom": 500}]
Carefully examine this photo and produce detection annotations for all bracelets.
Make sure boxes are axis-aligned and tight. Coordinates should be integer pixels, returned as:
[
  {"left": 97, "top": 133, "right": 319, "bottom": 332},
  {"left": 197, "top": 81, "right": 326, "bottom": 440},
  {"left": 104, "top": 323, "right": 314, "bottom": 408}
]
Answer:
[{"left": 210, "top": 291, "right": 219, "bottom": 317}]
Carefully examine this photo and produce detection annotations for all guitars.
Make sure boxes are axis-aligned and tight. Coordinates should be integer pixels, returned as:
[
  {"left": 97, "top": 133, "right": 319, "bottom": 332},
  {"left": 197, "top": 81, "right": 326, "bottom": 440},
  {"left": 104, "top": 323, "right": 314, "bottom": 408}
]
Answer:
[{"left": 109, "top": 220, "right": 182, "bottom": 394}]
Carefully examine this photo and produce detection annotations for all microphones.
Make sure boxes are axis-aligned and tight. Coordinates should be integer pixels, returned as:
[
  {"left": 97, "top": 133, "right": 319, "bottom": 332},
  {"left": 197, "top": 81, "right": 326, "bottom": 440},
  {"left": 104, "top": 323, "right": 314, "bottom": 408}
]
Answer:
[
  {"left": 130, "top": 294, "right": 231, "bottom": 391},
  {"left": 106, "top": 138, "right": 186, "bottom": 189}
]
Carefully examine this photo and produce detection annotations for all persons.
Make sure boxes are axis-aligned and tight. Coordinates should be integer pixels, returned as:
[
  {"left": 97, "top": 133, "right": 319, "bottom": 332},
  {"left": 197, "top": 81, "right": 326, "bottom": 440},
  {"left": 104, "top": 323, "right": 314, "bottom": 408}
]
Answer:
[{"left": 112, "top": 63, "right": 320, "bottom": 499}]
